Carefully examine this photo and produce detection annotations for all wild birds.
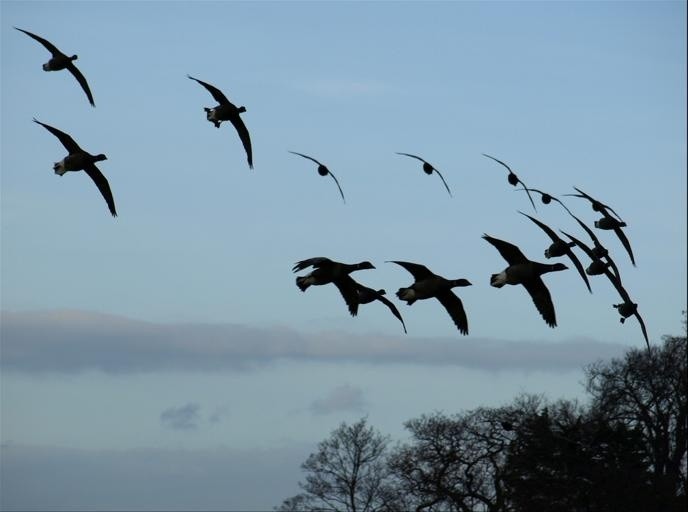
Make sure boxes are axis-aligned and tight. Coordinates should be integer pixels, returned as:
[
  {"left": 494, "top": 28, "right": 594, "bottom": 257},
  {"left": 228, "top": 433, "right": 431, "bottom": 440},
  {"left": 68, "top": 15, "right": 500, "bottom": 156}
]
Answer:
[
  {"left": 187, "top": 76, "right": 252, "bottom": 169},
  {"left": 384, "top": 260, "right": 473, "bottom": 336},
  {"left": 291, "top": 257, "right": 376, "bottom": 317},
  {"left": 356, "top": 283, "right": 408, "bottom": 334},
  {"left": 15, "top": 28, "right": 96, "bottom": 107},
  {"left": 288, "top": 151, "right": 344, "bottom": 200},
  {"left": 396, "top": 153, "right": 451, "bottom": 194},
  {"left": 481, "top": 153, "right": 651, "bottom": 361},
  {"left": 31, "top": 117, "right": 118, "bottom": 218}
]
